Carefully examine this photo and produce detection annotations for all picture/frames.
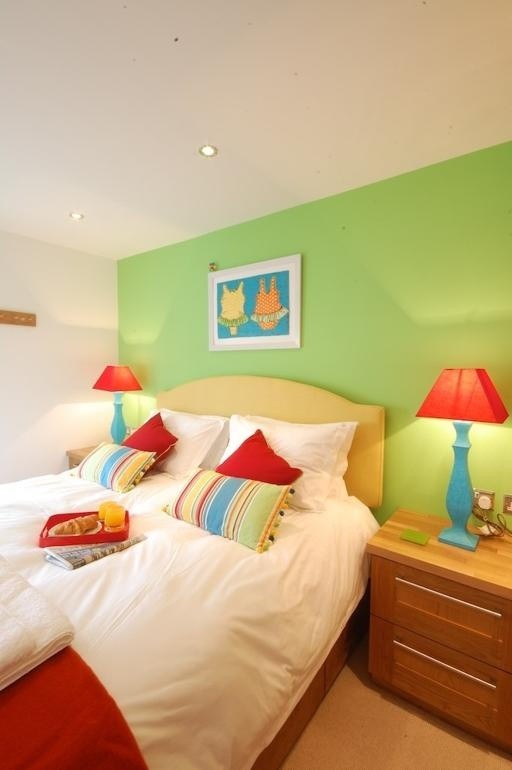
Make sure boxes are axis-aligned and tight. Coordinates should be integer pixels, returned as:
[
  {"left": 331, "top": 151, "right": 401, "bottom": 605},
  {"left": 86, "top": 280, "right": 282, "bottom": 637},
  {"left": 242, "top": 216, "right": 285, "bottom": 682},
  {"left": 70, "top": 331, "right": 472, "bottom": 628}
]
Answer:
[{"left": 207, "top": 252, "right": 302, "bottom": 353}]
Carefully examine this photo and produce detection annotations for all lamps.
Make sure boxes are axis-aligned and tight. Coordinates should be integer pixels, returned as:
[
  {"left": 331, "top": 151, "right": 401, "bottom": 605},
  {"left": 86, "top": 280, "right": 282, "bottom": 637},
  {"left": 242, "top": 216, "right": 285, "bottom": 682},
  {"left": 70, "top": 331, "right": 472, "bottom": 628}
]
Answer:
[
  {"left": 415, "top": 363, "right": 512, "bottom": 553},
  {"left": 92, "top": 364, "right": 143, "bottom": 444}
]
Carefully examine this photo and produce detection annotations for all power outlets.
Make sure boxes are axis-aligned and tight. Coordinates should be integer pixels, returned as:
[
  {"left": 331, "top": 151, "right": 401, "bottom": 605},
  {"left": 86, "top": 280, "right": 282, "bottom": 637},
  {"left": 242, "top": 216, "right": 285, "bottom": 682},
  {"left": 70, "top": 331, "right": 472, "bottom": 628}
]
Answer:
[
  {"left": 502, "top": 493, "right": 512, "bottom": 515},
  {"left": 474, "top": 487, "right": 497, "bottom": 516}
]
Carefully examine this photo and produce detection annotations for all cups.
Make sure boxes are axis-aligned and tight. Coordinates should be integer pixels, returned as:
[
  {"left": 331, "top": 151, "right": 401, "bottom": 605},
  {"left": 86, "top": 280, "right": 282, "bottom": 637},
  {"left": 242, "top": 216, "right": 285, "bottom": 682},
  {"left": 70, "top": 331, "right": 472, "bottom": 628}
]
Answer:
[
  {"left": 98, "top": 499, "right": 115, "bottom": 523},
  {"left": 104, "top": 504, "right": 125, "bottom": 531}
]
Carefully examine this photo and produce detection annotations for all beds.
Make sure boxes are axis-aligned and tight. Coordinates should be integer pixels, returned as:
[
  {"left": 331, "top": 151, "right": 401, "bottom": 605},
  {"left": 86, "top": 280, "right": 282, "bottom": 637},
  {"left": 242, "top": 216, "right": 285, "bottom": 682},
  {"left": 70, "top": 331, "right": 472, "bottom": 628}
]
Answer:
[{"left": 0, "top": 373, "right": 385, "bottom": 769}]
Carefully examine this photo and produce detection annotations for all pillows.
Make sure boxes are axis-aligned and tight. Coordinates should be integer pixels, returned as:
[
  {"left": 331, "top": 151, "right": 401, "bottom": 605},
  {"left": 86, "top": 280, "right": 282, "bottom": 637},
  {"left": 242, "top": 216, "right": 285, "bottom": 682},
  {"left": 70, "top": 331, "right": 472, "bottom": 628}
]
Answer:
[
  {"left": 215, "top": 428, "right": 305, "bottom": 487},
  {"left": 70, "top": 439, "right": 157, "bottom": 496},
  {"left": 161, "top": 467, "right": 295, "bottom": 554},
  {"left": 121, "top": 414, "right": 178, "bottom": 479},
  {"left": 218, "top": 414, "right": 359, "bottom": 513},
  {"left": 152, "top": 408, "right": 228, "bottom": 483}
]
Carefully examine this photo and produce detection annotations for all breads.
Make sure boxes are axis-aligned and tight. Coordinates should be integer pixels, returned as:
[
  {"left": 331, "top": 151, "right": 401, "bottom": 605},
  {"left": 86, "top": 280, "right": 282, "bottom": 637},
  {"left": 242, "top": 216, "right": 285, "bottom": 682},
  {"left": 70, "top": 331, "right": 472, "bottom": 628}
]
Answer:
[{"left": 48, "top": 513, "right": 99, "bottom": 538}]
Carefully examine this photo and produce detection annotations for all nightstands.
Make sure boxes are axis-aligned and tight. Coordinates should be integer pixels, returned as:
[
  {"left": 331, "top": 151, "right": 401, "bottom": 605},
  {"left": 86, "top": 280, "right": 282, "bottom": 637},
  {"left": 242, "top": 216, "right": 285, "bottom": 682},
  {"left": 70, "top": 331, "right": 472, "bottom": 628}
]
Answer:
[
  {"left": 361, "top": 513, "right": 511, "bottom": 758},
  {"left": 65, "top": 447, "right": 97, "bottom": 471}
]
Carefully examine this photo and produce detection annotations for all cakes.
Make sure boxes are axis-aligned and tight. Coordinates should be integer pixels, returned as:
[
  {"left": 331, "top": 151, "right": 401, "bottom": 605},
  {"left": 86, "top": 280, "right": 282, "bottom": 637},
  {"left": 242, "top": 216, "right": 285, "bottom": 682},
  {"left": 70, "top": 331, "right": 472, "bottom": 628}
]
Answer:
[
  {"left": 106, "top": 505, "right": 125, "bottom": 533},
  {"left": 98, "top": 501, "right": 116, "bottom": 524}
]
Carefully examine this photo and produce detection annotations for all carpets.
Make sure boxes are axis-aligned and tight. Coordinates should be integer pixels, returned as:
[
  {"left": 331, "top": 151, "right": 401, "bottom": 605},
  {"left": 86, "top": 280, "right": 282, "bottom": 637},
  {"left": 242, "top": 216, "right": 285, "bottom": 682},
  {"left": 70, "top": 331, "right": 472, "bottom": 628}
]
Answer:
[{"left": 279, "top": 641, "right": 512, "bottom": 770}]
